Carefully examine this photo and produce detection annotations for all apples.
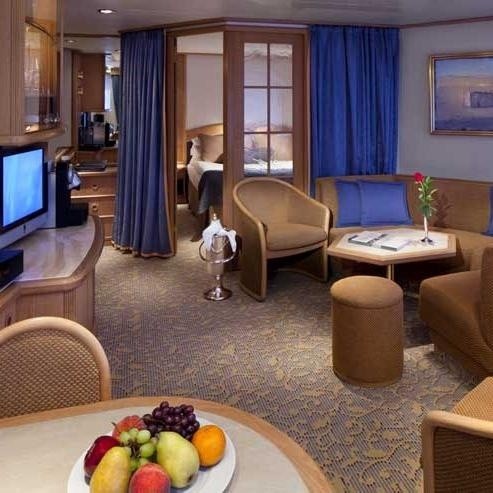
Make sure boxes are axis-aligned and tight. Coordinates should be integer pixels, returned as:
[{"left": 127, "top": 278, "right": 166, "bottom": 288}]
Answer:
[
  {"left": 110, "top": 415, "right": 143, "bottom": 438},
  {"left": 84, "top": 437, "right": 118, "bottom": 477},
  {"left": 127, "top": 464, "right": 171, "bottom": 493}
]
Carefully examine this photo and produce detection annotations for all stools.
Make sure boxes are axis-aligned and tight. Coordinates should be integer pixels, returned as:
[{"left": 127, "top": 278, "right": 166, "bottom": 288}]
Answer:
[{"left": 330, "top": 275, "right": 404, "bottom": 387}]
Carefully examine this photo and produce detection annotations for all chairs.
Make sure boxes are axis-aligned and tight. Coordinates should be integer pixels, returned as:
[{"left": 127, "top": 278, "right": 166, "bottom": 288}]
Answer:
[
  {"left": 422, "top": 375, "right": 493, "bottom": 493},
  {"left": 1, "top": 316, "right": 112, "bottom": 420},
  {"left": 232, "top": 177, "right": 331, "bottom": 303}
]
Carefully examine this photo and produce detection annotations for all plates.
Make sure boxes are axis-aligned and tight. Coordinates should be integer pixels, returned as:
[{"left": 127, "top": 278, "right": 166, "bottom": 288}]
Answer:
[{"left": 67, "top": 414, "right": 237, "bottom": 493}]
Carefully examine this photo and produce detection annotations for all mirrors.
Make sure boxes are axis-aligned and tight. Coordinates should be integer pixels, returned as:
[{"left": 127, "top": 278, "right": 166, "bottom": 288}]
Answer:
[{"left": 54, "top": 33, "right": 121, "bottom": 167}]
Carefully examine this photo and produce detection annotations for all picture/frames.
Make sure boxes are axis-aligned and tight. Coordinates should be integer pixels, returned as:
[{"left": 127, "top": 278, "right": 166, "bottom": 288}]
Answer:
[{"left": 428, "top": 50, "right": 493, "bottom": 136}]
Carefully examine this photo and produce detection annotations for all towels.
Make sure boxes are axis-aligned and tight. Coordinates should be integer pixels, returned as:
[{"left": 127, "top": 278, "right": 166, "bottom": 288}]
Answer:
[{"left": 203, "top": 220, "right": 236, "bottom": 252}]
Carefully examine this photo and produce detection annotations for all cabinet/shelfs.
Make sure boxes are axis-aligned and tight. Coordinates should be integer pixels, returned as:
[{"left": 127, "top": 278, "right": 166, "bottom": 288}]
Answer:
[
  {"left": 81, "top": 54, "right": 111, "bottom": 113},
  {"left": 71, "top": 167, "right": 117, "bottom": 241},
  {"left": 76, "top": 144, "right": 118, "bottom": 162},
  {"left": 1, "top": 215, "right": 105, "bottom": 337}
]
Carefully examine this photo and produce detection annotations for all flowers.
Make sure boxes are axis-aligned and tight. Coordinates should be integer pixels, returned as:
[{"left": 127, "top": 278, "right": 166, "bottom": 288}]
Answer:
[{"left": 414, "top": 172, "right": 438, "bottom": 217}]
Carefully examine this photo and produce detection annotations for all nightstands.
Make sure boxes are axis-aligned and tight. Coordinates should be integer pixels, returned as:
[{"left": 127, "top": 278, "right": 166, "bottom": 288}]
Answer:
[{"left": 177, "top": 163, "right": 187, "bottom": 203}]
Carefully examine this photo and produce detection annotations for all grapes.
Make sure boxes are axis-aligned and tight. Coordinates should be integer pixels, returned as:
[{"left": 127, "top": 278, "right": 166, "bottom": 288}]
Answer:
[
  {"left": 143, "top": 400, "right": 201, "bottom": 440},
  {"left": 118, "top": 425, "right": 154, "bottom": 473}
]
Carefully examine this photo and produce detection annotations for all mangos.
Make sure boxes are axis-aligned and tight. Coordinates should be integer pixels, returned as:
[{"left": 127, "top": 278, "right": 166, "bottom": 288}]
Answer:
[{"left": 90, "top": 448, "right": 128, "bottom": 493}]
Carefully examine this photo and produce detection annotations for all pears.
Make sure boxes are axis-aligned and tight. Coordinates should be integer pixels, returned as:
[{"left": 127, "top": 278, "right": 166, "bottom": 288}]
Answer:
[{"left": 154, "top": 432, "right": 198, "bottom": 488}]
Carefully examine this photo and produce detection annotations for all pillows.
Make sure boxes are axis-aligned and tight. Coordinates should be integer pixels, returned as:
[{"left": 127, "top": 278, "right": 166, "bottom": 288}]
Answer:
[
  {"left": 335, "top": 178, "right": 362, "bottom": 227},
  {"left": 481, "top": 186, "right": 493, "bottom": 236},
  {"left": 199, "top": 133, "right": 253, "bottom": 162},
  {"left": 357, "top": 179, "right": 416, "bottom": 228},
  {"left": 253, "top": 128, "right": 293, "bottom": 160}
]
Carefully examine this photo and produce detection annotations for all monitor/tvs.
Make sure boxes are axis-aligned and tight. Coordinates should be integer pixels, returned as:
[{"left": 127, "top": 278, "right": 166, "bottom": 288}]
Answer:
[{"left": 0, "top": 141, "right": 48, "bottom": 250}]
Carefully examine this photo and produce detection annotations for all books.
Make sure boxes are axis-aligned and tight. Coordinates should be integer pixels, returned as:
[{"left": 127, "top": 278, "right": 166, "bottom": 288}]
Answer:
[{"left": 348, "top": 231, "right": 410, "bottom": 251}]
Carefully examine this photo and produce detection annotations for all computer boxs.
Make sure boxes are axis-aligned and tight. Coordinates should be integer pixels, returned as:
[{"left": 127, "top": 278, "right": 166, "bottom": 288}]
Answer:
[{"left": 37, "top": 160, "right": 70, "bottom": 229}]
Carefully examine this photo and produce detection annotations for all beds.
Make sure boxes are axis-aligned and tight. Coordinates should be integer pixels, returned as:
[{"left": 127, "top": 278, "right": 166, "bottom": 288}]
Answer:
[{"left": 186, "top": 124, "right": 293, "bottom": 232}]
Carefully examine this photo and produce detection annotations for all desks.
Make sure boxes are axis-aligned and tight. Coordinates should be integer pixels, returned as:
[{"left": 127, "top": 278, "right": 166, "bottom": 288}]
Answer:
[{"left": 1, "top": 397, "right": 332, "bottom": 493}]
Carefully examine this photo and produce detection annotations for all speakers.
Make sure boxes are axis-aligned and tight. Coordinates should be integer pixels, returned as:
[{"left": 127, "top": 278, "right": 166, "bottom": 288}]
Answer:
[
  {"left": 63, "top": 202, "right": 89, "bottom": 227},
  {"left": 0, "top": 249, "right": 24, "bottom": 290}
]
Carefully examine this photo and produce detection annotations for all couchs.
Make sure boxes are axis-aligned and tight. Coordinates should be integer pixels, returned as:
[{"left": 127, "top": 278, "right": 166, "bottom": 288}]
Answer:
[{"left": 316, "top": 174, "right": 493, "bottom": 380}]
